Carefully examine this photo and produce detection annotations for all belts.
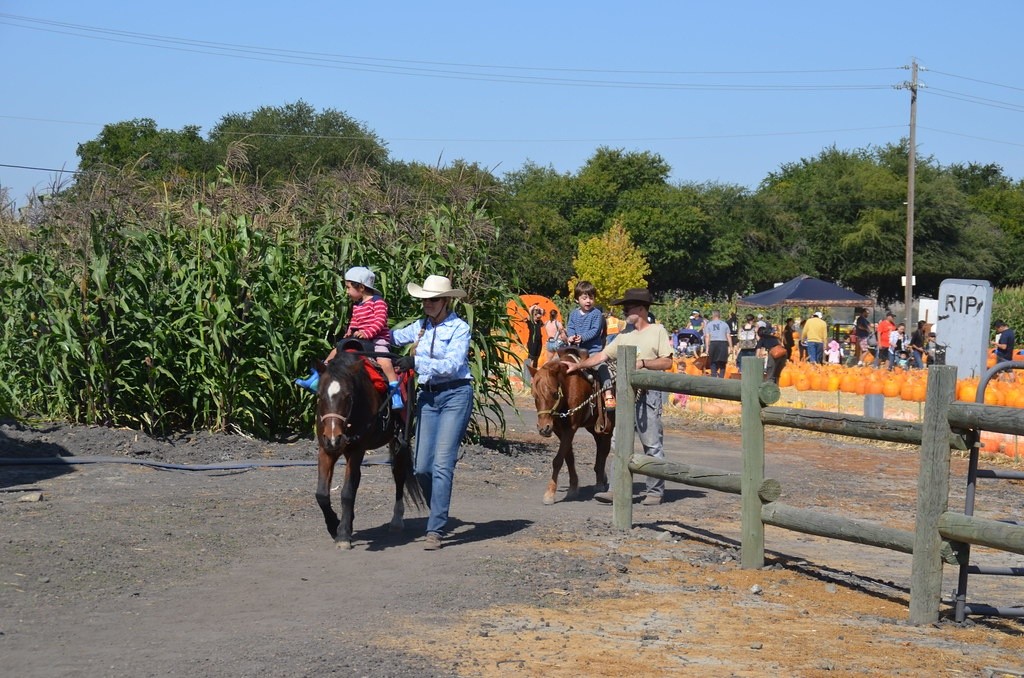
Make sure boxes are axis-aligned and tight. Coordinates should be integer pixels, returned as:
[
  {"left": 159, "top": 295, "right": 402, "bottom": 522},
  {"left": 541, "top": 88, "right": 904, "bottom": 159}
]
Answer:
[{"left": 425, "top": 380, "right": 469, "bottom": 393}]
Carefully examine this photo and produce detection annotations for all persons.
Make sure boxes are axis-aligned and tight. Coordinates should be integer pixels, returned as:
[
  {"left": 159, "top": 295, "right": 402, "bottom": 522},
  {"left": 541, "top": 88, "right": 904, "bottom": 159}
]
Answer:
[
  {"left": 294, "top": 267, "right": 404, "bottom": 410},
  {"left": 391, "top": 274, "right": 474, "bottom": 549},
  {"left": 676, "top": 309, "right": 936, "bottom": 383},
  {"left": 560, "top": 287, "right": 674, "bottom": 504},
  {"left": 989, "top": 319, "right": 1015, "bottom": 380},
  {"left": 527, "top": 305, "right": 565, "bottom": 370},
  {"left": 566, "top": 281, "right": 615, "bottom": 411}
]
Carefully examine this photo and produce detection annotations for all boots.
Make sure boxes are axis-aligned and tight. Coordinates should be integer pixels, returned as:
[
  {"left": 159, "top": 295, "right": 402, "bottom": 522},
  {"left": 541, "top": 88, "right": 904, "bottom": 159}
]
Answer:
[
  {"left": 295, "top": 368, "right": 321, "bottom": 394},
  {"left": 390, "top": 383, "right": 404, "bottom": 410}
]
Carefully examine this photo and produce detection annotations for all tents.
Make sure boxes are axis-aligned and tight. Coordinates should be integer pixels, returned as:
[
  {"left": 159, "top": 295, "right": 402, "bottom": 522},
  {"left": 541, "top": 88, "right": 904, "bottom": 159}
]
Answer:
[{"left": 733, "top": 274, "right": 875, "bottom": 337}]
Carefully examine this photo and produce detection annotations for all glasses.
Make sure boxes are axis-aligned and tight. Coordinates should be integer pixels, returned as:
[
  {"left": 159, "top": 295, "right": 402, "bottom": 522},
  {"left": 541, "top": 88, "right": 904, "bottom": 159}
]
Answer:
[
  {"left": 622, "top": 304, "right": 647, "bottom": 313},
  {"left": 422, "top": 297, "right": 446, "bottom": 303}
]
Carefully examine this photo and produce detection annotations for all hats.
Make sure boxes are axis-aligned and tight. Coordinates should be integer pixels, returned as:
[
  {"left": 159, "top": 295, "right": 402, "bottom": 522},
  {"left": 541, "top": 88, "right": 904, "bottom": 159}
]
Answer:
[
  {"left": 345, "top": 267, "right": 382, "bottom": 295},
  {"left": 887, "top": 312, "right": 896, "bottom": 317},
  {"left": 813, "top": 311, "right": 823, "bottom": 319},
  {"left": 757, "top": 313, "right": 763, "bottom": 318},
  {"left": 614, "top": 288, "right": 665, "bottom": 305},
  {"left": 828, "top": 340, "right": 839, "bottom": 350},
  {"left": 928, "top": 332, "right": 936, "bottom": 337},
  {"left": 407, "top": 275, "right": 467, "bottom": 299}
]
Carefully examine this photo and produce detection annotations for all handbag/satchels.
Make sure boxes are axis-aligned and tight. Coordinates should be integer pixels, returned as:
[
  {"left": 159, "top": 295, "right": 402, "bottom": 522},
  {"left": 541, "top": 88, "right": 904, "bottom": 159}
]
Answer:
[{"left": 769, "top": 344, "right": 788, "bottom": 359}]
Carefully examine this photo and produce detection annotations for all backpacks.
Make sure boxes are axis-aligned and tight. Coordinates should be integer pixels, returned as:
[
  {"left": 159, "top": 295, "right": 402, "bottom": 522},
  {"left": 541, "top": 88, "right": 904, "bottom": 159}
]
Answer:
[{"left": 737, "top": 325, "right": 757, "bottom": 349}]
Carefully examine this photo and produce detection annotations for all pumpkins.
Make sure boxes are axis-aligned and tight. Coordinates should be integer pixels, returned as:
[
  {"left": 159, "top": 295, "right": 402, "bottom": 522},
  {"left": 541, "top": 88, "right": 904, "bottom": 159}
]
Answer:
[{"left": 481, "top": 340, "right": 1024, "bottom": 458}]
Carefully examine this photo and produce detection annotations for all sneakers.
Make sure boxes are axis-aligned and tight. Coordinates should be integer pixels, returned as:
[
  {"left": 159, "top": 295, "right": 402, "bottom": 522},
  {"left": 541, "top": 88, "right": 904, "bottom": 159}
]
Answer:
[
  {"left": 423, "top": 533, "right": 441, "bottom": 549},
  {"left": 642, "top": 493, "right": 664, "bottom": 505},
  {"left": 604, "top": 395, "right": 616, "bottom": 409},
  {"left": 594, "top": 491, "right": 613, "bottom": 506}
]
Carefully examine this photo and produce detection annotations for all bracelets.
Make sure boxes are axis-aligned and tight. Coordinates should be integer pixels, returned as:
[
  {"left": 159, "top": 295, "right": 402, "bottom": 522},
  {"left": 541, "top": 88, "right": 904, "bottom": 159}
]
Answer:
[{"left": 641, "top": 359, "right": 645, "bottom": 367}]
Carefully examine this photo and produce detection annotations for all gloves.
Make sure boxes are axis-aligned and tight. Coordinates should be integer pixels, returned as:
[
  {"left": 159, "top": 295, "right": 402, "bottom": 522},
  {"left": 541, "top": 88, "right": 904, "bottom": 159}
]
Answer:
[{"left": 394, "top": 356, "right": 415, "bottom": 375}]
